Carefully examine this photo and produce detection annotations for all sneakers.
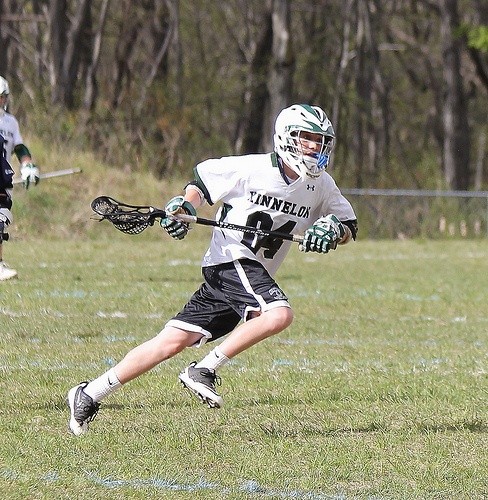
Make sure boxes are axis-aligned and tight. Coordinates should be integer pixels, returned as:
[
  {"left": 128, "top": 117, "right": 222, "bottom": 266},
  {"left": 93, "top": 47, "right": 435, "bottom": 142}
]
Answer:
[
  {"left": 66, "top": 381, "right": 102, "bottom": 436},
  {"left": 0, "top": 261, "right": 18, "bottom": 281},
  {"left": 178, "top": 361, "right": 223, "bottom": 409}
]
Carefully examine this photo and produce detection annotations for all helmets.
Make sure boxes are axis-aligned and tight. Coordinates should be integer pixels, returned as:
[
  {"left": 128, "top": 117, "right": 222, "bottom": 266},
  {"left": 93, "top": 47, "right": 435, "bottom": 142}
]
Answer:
[
  {"left": 0, "top": 75, "right": 9, "bottom": 117},
  {"left": 272, "top": 103, "right": 336, "bottom": 180}
]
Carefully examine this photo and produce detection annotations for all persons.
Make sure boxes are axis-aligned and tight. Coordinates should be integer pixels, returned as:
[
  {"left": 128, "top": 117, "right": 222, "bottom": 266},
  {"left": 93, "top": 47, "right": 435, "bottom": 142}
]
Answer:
[
  {"left": 68, "top": 104, "right": 359, "bottom": 439},
  {"left": 0, "top": 75, "right": 40, "bottom": 282}
]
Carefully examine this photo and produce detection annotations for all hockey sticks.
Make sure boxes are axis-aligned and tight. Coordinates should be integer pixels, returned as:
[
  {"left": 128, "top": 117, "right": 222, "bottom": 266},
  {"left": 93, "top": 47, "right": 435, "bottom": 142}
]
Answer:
[
  {"left": 12, "top": 168, "right": 83, "bottom": 185},
  {"left": 87, "top": 194, "right": 338, "bottom": 251}
]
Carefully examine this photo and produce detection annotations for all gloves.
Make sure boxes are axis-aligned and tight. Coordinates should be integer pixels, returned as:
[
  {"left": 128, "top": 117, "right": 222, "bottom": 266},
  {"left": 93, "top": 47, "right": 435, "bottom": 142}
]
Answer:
[
  {"left": 159, "top": 195, "right": 196, "bottom": 241},
  {"left": 20, "top": 163, "right": 40, "bottom": 189},
  {"left": 298, "top": 214, "right": 345, "bottom": 253}
]
